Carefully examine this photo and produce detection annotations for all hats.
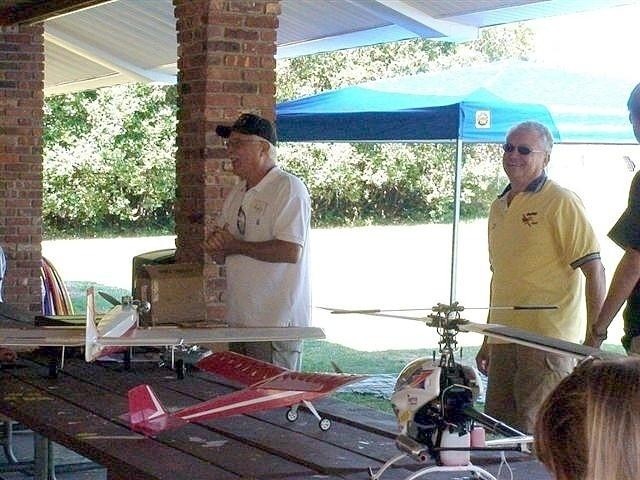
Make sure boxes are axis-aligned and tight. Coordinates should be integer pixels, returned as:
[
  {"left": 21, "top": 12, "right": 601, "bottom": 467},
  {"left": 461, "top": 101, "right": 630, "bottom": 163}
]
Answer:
[{"left": 216, "top": 113, "right": 277, "bottom": 146}]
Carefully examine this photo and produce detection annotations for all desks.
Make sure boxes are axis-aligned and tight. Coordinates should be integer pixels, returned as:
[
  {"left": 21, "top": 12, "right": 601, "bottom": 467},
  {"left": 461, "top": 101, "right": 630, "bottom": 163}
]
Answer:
[{"left": 0, "top": 303, "right": 555, "bottom": 480}]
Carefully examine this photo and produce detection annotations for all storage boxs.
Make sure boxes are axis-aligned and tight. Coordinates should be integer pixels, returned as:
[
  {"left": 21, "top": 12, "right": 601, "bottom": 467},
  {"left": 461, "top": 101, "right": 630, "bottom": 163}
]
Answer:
[{"left": 136, "top": 264, "right": 228, "bottom": 352}]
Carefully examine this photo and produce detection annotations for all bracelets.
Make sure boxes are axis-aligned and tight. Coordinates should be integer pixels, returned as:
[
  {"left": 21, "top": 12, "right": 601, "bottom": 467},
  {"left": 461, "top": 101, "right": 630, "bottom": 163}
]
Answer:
[{"left": 591, "top": 324, "right": 607, "bottom": 342}]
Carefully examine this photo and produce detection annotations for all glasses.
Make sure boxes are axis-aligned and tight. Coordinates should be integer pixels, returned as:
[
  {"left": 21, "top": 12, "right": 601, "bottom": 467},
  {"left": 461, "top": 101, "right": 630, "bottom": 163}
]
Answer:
[
  {"left": 223, "top": 139, "right": 264, "bottom": 149},
  {"left": 503, "top": 144, "right": 543, "bottom": 155},
  {"left": 237, "top": 207, "right": 246, "bottom": 235}
]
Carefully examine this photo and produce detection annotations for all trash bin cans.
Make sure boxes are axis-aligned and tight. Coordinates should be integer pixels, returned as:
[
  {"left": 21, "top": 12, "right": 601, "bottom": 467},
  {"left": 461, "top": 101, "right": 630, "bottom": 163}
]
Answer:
[{"left": 132, "top": 249, "right": 176, "bottom": 326}]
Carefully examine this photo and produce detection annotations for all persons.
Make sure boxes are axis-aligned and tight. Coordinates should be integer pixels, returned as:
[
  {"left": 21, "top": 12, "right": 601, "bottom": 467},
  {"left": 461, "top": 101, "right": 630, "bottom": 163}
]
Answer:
[
  {"left": 533, "top": 356, "right": 640, "bottom": 480},
  {"left": 199, "top": 112, "right": 313, "bottom": 372},
  {"left": 582, "top": 81, "right": 640, "bottom": 356},
  {"left": 474, "top": 121, "right": 606, "bottom": 452}
]
